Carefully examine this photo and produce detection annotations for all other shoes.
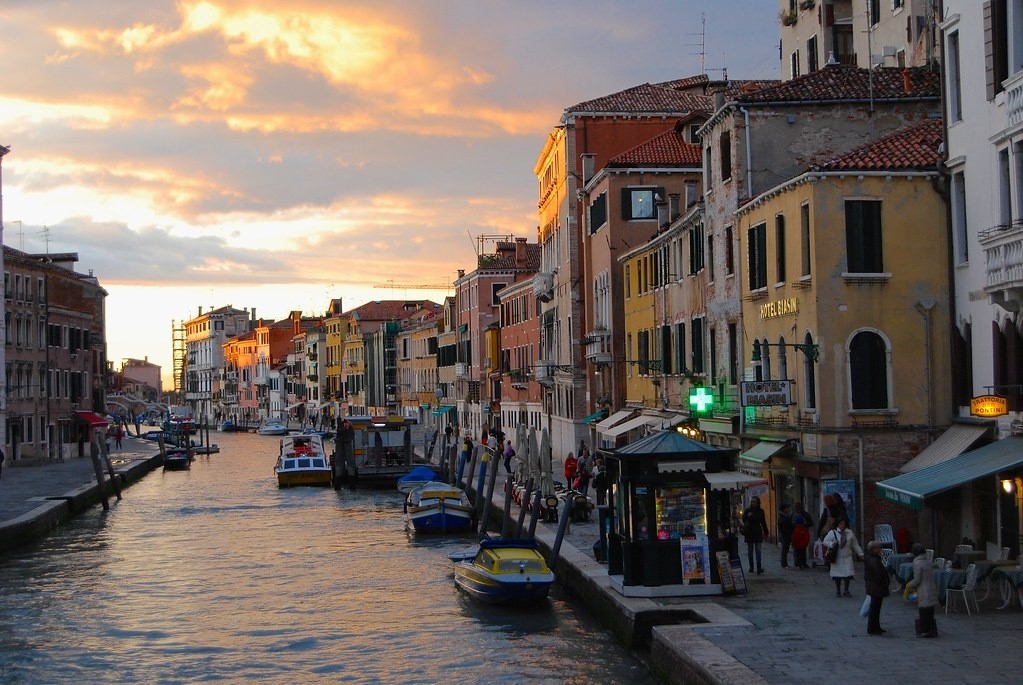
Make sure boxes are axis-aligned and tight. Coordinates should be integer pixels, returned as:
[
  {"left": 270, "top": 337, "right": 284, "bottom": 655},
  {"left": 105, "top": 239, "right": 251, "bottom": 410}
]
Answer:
[
  {"left": 749, "top": 567, "right": 753, "bottom": 572},
  {"left": 757, "top": 569, "right": 764, "bottom": 575},
  {"left": 868, "top": 628, "right": 886, "bottom": 635},
  {"left": 844, "top": 591, "right": 852, "bottom": 597},
  {"left": 836, "top": 591, "right": 841, "bottom": 597},
  {"left": 917, "top": 631, "right": 936, "bottom": 638}
]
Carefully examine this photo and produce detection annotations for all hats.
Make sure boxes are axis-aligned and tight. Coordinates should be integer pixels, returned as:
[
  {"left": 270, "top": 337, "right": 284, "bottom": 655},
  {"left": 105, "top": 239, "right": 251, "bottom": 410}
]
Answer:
[
  {"left": 779, "top": 504, "right": 791, "bottom": 511},
  {"left": 793, "top": 517, "right": 804, "bottom": 524}
]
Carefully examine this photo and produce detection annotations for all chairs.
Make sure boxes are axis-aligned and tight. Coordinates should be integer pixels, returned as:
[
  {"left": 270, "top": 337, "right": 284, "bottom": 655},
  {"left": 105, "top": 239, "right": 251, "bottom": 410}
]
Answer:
[
  {"left": 873, "top": 524, "right": 897, "bottom": 555},
  {"left": 944, "top": 564, "right": 981, "bottom": 615},
  {"left": 925, "top": 544, "right": 974, "bottom": 569},
  {"left": 879, "top": 548, "right": 894, "bottom": 582}
]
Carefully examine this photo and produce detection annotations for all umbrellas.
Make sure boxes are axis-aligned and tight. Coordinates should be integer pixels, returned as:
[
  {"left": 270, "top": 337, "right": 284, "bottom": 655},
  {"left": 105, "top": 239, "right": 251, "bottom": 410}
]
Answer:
[{"left": 516, "top": 424, "right": 555, "bottom": 497}]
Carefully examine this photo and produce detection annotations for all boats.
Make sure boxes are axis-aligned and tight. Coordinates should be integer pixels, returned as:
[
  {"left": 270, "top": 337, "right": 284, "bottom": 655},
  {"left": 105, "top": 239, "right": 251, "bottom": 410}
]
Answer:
[
  {"left": 397, "top": 466, "right": 476, "bottom": 534},
  {"left": 256, "top": 412, "right": 425, "bottom": 487},
  {"left": 448, "top": 537, "right": 555, "bottom": 604},
  {"left": 147, "top": 417, "right": 221, "bottom": 469},
  {"left": 218, "top": 422, "right": 237, "bottom": 431}
]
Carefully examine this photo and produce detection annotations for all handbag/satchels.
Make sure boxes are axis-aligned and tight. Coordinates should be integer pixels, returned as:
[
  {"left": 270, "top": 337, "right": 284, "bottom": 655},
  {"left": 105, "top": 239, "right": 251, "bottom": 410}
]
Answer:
[
  {"left": 814, "top": 537, "right": 826, "bottom": 561},
  {"left": 915, "top": 618, "right": 938, "bottom": 637},
  {"left": 824, "top": 543, "right": 839, "bottom": 563},
  {"left": 860, "top": 594, "right": 871, "bottom": 618},
  {"left": 820, "top": 507, "right": 834, "bottom": 536}
]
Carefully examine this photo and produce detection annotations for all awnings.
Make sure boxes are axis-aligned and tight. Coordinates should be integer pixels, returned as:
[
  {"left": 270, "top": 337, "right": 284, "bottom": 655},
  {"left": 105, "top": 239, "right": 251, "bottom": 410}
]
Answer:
[
  {"left": 875, "top": 435, "right": 1023, "bottom": 511},
  {"left": 595, "top": 411, "right": 635, "bottom": 433},
  {"left": 738, "top": 441, "right": 793, "bottom": 464},
  {"left": 285, "top": 403, "right": 304, "bottom": 410},
  {"left": 582, "top": 410, "right": 606, "bottom": 428},
  {"left": 73, "top": 412, "right": 108, "bottom": 429},
  {"left": 658, "top": 460, "right": 706, "bottom": 474},
  {"left": 602, "top": 416, "right": 657, "bottom": 443},
  {"left": 652, "top": 414, "right": 691, "bottom": 432},
  {"left": 309, "top": 413, "right": 342, "bottom": 430},
  {"left": 703, "top": 471, "right": 767, "bottom": 491},
  {"left": 432, "top": 406, "right": 455, "bottom": 416},
  {"left": 897, "top": 422, "right": 988, "bottom": 474}
]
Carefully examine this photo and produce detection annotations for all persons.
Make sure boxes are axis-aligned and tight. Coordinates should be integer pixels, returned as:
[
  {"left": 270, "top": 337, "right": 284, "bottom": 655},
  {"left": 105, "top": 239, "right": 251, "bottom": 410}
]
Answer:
[
  {"left": 907, "top": 543, "right": 944, "bottom": 638},
  {"left": 115, "top": 430, "right": 122, "bottom": 451},
  {"left": 864, "top": 541, "right": 894, "bottom": 636},
  {"left": 778, "top": 502, "right": 813, "bottom": 570},
  {"left": 445, "top": 422, "right": 516, "bottom": 474},
  {"left": 591, "top": 458, "right": 606, "bottom": 479},
  {"left": 578, "top": 440, "right": 590, "bottom": 459},
  {"left": 823, "top": 518, "right": 863, "bottom": 598},
  {"left": 105, "top": 433, "right": 111, "bottom": 455},
  {"left": 216, "top": 411, "right": 250, "bottom": 423},
  {"left": 564, "top": 452, "right": 577, "bottom": 490},
  {"left": 593, "top": 466, "right": 607, "bottom": 506},
  {"left": 742, "top": 496, "right": 769, "bottom": 574},
  {"left": 576, "top": 448, "right": 593, "bottom": 496}
]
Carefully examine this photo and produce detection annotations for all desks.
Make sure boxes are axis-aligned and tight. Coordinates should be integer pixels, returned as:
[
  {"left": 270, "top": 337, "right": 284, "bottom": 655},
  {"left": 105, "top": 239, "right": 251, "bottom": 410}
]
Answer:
[
  {"left": 887, "top": 554, "right": 914, "bottom": 583},
  {"left": 994, "top": 563, "right": 1023, "bottom": 611},
  {"left": 932, "top": 569, "right": 966, "bottom": 605},
  {"left": 891, "top": 564, "right": 914, "bottom": 592}
]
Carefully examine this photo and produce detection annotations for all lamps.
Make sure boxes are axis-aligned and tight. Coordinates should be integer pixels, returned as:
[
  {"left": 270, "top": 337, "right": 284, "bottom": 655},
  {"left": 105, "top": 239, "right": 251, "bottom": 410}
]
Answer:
[
  {"left": 750, "top": 338, "right": 820, "bottom": 366},
  {"left": 526, "top": 365, "right": 573, "bottom": 376},
  {"left": 595, "top": 359, "right": 664, "bottom": 373}
]
